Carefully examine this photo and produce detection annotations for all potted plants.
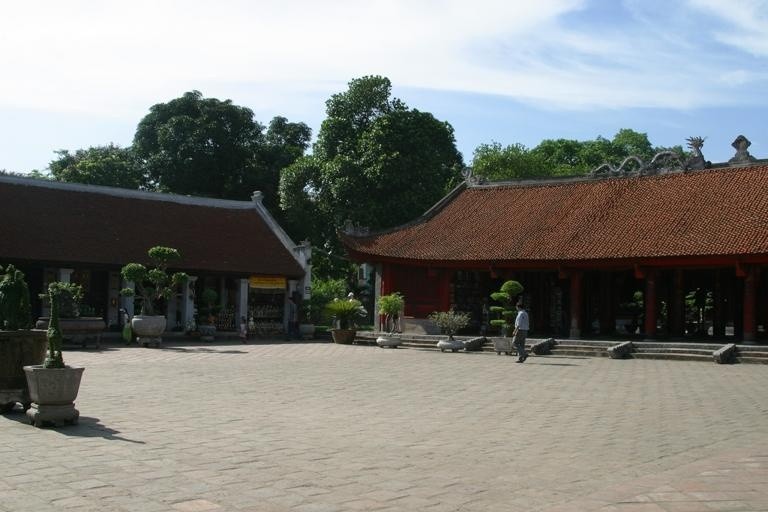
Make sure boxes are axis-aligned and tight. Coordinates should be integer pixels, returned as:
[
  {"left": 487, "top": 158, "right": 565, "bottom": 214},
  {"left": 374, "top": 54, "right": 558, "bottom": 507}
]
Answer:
[
  {"left": 22, "top": 282, "right": 85, "bottom": 427},
  {"left": 427, "top": 306, "right": 470, "bottom": 352},
  {"left": 0, "top": 263, "right": 47, "bottom": 415},
  {"left": 119, "top": 246, "right": 189, "bottom": 346},
  {"left": 375, "top": 294, "right": 403, "bottom": 347},
  {"left": 321, "top": 297, "right": 368, "bottom": 344},
  {"left": 490, "top": 279, "right": 524, "bottom": 356},
  {"left": 685, "top": 288, "right": 715, "bottom": 342},
  {"left": 656, "top": 301, "right": 670, "bottom": 339},
  {"left": 197, "top": 289, "right": 218, "bottom": 341},
  {"left": 36, "top": 280, "right": 106, "bottom": 349},
  {"left": 298, "top": 297, "right": 320, "bottom": 340}
]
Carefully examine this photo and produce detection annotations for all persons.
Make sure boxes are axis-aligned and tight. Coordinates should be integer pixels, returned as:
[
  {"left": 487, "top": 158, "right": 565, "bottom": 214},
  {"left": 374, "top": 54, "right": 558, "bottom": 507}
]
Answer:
[
  {"left": 511, "top": 301, "right": 529, "bottom": 365},
  {"left": 239, "top": 316, "right": 247, "bottom": 344}
]
